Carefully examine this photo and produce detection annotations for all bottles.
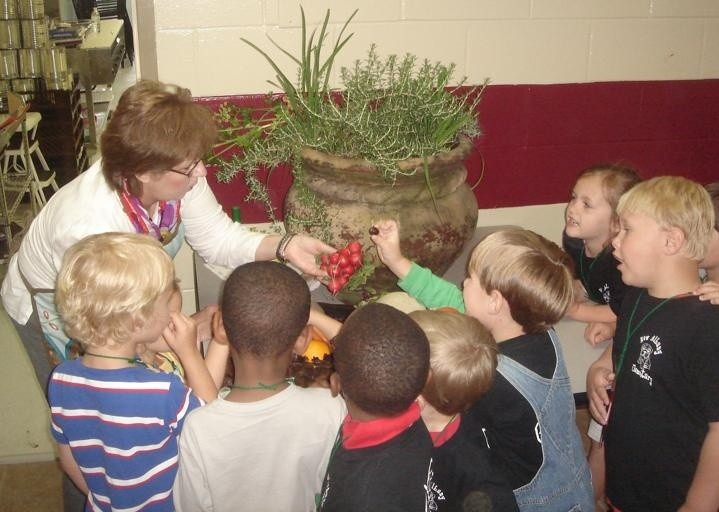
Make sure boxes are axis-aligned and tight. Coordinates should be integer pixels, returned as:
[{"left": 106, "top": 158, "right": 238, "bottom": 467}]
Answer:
[{"left": 91, "top": 7, "right": 100, "bottom": 33}]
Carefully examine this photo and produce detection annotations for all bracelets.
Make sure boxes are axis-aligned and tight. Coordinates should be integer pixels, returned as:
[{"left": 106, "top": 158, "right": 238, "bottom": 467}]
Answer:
[{"left": 276, "top": 232, "right": 298, "bottom": 263}]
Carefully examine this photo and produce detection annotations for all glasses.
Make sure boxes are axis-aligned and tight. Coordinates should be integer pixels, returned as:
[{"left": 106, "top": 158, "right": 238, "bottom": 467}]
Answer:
[{"left": 167, "top": 159, "right": 201, "bottom": 178}]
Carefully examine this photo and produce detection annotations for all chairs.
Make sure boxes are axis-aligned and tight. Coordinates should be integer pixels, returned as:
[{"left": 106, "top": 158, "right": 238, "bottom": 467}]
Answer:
[{"left": 1, "top": 90, "right": 61, "bottom": 263}]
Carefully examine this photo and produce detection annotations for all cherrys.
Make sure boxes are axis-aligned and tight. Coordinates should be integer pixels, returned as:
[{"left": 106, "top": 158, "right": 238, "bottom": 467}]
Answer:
[{"left": 320, "top": 241, "right": 364, "bottom": 294}]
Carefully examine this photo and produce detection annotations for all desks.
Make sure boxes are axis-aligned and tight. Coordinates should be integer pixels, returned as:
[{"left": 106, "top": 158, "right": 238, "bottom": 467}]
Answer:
[{"left": 191, "top": 202, "right": 615, "bottom": 410}]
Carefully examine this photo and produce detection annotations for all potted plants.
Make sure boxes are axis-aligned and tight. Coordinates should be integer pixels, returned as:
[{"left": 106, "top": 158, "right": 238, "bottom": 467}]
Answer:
[{"left": 203, "top": 4, "right": 490, "bottom": 305}]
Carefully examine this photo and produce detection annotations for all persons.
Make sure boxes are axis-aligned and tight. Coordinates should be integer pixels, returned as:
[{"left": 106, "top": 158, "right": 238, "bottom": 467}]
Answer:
[
  {"left": 372, "top": 220, "right": 595, "bottom": 512},
  {"left": 139, "top": 282, "right": 230, "bottom": 391},
  {"left": 171, "top": 260, "right": 349, "bottom": 512},
  {"left": 559, "top": 164, "right": 719, "bottom": 512},
  {"left": 319, "top": 300, "right": 434, "bottom": 511},
  {"left": 44, "top": 232, "right": 219, "bottom": 512},
  {"left": 307, "top": 306, "right": 517, "bottom": 511},
  {"left": 0, "top": 77, "right": 338, "bottom": 512}
]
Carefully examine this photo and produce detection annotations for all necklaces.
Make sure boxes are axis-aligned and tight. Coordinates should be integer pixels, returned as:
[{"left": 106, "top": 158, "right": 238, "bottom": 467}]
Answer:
[
  {"left": 231, "top": 380, "right": 290, "bottom": 392},
  {"left": 85, "top": 351, "right": 135, "bottom": 363}
]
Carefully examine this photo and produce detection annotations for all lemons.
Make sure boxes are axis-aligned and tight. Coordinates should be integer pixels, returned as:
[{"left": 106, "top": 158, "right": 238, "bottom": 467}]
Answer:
[{"left": 301, "top": 339, "right": 331, "bottom": 363}]
